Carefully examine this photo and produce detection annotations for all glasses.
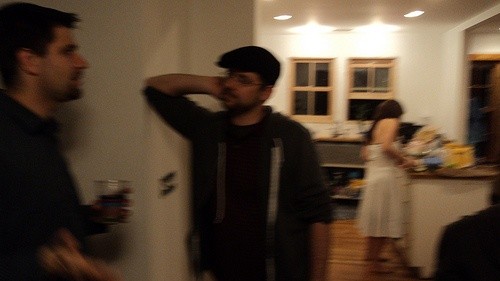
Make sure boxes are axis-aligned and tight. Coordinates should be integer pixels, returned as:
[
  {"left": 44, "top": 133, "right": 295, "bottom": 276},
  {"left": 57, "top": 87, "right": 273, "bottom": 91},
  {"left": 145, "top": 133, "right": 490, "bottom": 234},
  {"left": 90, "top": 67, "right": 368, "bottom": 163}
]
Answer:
[{"left": 226, "top": 71, "right": 265, "bottom": 88}]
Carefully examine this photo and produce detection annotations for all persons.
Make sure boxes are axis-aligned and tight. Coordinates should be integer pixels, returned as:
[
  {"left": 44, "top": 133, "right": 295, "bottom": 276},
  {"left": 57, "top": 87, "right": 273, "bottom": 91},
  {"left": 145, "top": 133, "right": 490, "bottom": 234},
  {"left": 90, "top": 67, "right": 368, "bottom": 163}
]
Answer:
[
  {"left": 140, "top": 46, "right": 334, "bottom": 281},
  {"left": 1, "top": 1, "right": 134, "bottom": 281},
  {"left": 427, "top": 161, "right": 500, "bottom": 281},
  {"left": 359, "top": 99, "right": 421, "bottom": 262}
]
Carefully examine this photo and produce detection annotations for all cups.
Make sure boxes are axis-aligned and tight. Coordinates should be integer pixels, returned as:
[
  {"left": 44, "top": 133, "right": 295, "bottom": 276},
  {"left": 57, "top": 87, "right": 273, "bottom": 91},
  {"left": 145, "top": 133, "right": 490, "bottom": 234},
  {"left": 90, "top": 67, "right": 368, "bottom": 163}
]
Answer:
[{"left": 94, "top": 181, "right": 128, "bottom": 223}]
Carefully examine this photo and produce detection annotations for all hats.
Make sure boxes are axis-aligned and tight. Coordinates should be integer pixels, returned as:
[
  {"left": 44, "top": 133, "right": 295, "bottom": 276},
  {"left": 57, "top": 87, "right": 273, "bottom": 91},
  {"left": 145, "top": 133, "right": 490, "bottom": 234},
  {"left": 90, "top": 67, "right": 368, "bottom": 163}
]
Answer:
[{"left": 216, "top": 45, "right": 280, "bottom": 84}]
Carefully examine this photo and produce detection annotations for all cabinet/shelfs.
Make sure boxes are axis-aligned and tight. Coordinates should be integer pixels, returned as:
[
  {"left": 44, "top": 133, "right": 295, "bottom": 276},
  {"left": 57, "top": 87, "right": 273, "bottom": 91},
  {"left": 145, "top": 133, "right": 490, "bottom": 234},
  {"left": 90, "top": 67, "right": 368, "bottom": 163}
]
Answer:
[{"left": 313, "top": 138, "right": 500, "bottom": 281}]
